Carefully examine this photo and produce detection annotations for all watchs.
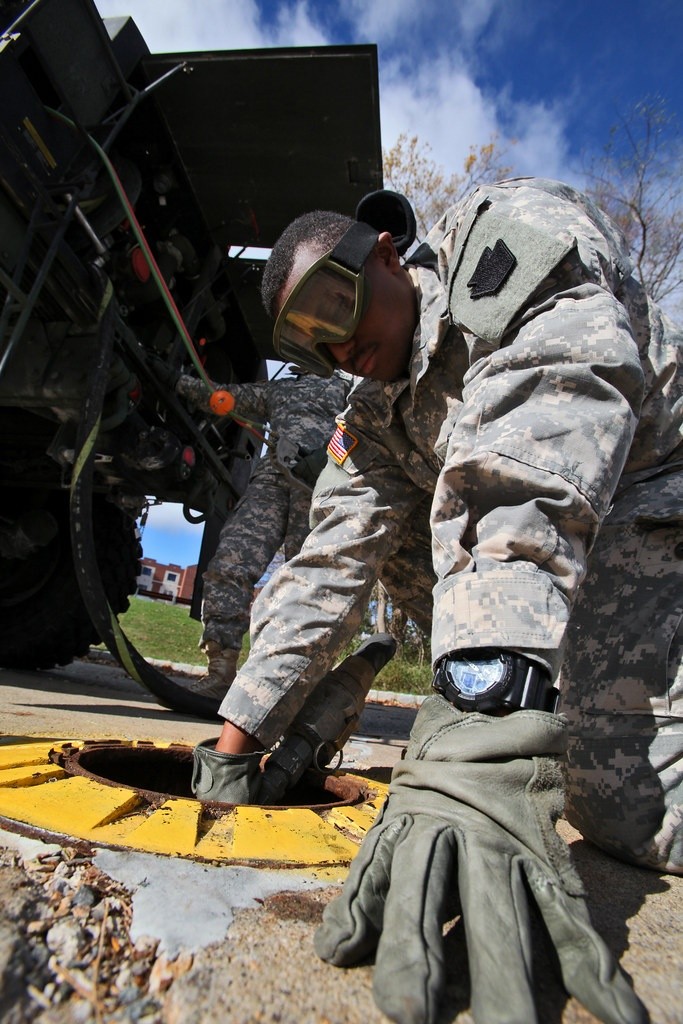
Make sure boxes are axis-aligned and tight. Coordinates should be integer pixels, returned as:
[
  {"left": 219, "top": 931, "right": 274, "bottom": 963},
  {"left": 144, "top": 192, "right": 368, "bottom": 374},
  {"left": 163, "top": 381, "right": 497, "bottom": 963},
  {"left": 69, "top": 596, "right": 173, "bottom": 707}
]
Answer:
[{"left": 430, "top": 648, "right": 563, "bottom": 717}]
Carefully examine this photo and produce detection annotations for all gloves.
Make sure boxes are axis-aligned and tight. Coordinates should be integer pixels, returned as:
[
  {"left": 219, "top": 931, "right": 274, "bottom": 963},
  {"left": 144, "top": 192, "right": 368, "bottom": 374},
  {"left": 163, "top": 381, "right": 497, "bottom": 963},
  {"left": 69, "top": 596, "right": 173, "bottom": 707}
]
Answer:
[
  {"left": 142, "top": 354, "right": 181, "bottom": 393},
  {"left": 191, "top": 737, "right": 265, "bottom": 803},
  {"left": 313, "top": 690, "right": 649, "bottom": 1024}
]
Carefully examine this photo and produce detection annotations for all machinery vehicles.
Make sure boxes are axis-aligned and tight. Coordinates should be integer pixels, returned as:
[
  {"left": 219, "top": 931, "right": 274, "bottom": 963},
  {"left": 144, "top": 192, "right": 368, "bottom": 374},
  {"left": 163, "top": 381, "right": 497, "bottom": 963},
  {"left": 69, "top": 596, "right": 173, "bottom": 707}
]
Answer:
[{"left": 0, "top": 0, "right": 419, "bottom": 724}]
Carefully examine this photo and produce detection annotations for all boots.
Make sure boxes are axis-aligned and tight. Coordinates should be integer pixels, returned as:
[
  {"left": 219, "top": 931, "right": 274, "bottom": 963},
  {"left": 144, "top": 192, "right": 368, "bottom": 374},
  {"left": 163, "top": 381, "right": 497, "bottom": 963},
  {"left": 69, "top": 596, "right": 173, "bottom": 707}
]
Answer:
[{"left": 182, "top": 643, "right": 239, "bottom": 701}]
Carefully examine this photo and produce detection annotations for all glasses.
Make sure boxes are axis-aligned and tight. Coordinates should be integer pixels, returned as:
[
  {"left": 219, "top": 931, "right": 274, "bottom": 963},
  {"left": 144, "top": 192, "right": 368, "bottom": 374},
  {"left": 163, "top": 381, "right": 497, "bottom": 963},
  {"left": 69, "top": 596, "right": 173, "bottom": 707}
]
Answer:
[{"left": 271, "top": 258, "right": 362, "bottom": 375}]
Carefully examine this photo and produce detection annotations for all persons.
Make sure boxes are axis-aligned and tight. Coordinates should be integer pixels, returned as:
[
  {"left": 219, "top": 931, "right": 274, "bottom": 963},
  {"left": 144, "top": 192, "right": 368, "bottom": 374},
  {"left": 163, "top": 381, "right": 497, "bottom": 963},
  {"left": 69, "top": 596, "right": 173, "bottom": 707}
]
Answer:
[
  {"left": 188, "top": 175, "right": 682, "bottom": 1024},
  {"left": 146, "top": 352, "right": 349, "bottom": 703}
]
